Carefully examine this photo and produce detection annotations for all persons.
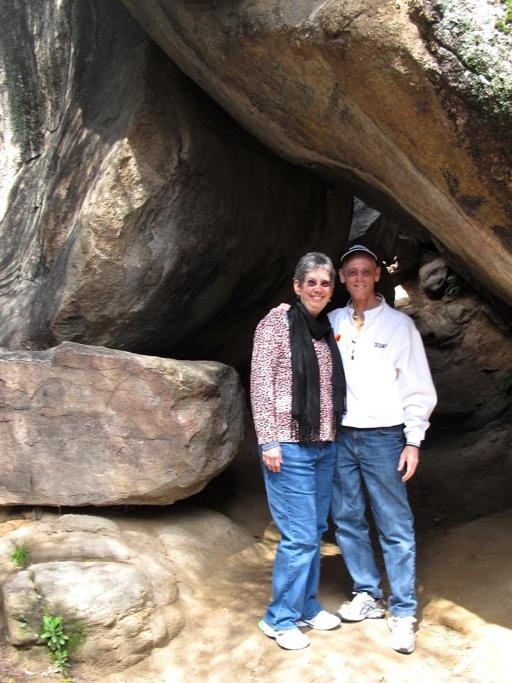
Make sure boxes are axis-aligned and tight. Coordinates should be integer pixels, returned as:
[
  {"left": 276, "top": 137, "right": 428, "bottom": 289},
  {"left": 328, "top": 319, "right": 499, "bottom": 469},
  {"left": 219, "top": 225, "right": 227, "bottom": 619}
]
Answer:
[
  {"left": 247, "top": 250, "right": 350, "bottom": 651},
  {"left": 322, "top": 244, "right": 440, "bottom": 655}
]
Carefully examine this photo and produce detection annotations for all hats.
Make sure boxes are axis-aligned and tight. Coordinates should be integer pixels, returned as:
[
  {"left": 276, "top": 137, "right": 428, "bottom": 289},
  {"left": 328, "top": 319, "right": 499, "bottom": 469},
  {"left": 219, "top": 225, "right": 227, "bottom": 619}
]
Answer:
[{"left": 340, "top": 245, "right": 377, "bottom": 263}]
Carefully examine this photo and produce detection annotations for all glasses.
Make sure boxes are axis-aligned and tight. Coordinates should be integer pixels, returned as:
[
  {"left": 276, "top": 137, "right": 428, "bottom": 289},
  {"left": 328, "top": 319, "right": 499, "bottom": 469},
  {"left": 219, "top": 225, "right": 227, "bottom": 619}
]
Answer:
[
  {"left": 306, "top": 280, "right": 329, "bottom": 287},
  {"left": 347, "top": 268, "right": 372, "bottom": 276}
]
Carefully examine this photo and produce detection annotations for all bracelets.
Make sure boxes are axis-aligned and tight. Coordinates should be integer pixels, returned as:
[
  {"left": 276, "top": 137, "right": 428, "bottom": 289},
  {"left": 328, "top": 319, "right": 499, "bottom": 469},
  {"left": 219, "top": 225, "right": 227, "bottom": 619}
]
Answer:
[{"left": 261, "top": 440, "right": 278, "bottom": 450}]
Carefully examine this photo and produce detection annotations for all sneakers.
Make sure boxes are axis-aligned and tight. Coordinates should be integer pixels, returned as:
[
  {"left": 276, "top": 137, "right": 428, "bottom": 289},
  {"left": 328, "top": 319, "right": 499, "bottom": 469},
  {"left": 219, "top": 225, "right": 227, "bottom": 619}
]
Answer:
[
  {"left": 387, "top": 616, "right": 417, "bottom": 653},
  {"left": 258, "top": 610, "right": 341, "bottom": 650},
  {"left": 339, "top": 592, "right": 385, "bottom": 621}
]
